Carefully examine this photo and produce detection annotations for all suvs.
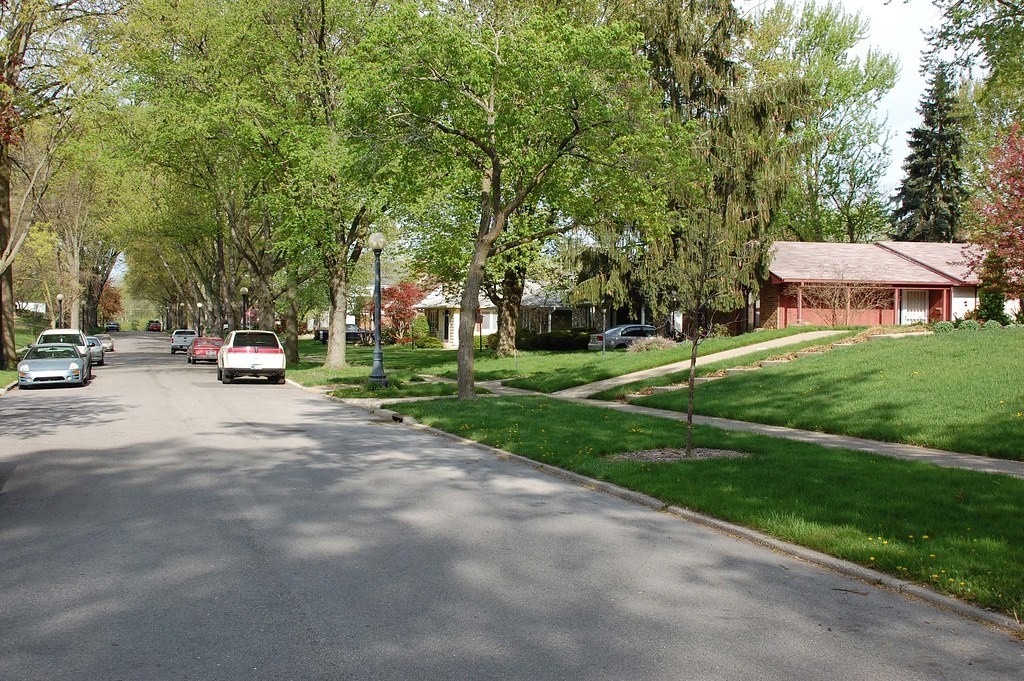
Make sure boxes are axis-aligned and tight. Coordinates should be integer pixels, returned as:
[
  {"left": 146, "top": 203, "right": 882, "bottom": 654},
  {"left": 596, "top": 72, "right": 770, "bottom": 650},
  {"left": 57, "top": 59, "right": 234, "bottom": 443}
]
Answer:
[
  {"left": 146, "top": 320, "right": 160, "bottom": 331},
  {"left": 36, "top": 328, "right": 96, "bottom": 380}
]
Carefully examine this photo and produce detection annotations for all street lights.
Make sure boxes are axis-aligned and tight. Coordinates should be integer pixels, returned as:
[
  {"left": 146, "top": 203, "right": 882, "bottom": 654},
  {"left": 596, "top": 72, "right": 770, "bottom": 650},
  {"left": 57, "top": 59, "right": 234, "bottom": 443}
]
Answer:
[
  {"left": 180, "top": 302, "right": 185, "bottom": 329},
  {"left": 81, "top": 300, "right": 86, "bottom": 334},
  {"left": 57, "top": 293, "right": 65, "bottom": 329},
  {"left": 166, "top": 307, "right": 170, "bottom": 331},
  {"left": 240, "top": 287, "right": 250, "bottom": 331},
  {"left": 367, "top": 232, "right": 391, "bottom": 389},
  {"left": 197, "top": 302, "right": 204, "bottom": 337}
]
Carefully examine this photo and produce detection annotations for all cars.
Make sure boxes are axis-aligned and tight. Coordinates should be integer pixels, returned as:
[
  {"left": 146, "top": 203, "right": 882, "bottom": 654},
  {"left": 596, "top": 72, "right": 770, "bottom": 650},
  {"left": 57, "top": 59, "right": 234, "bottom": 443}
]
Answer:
[
  {"left": 94, "top": 334, "right": 115, "bottom": 351},
  {"left": 16, "top": 343, "right": 88, "bottom": 389},
  {"left": 86, "top": 337, "right": 108, "bottom": 366},
  {"left": 587, "top": 325, "right": 663, "bottom": 353},
  {"left": 314, "top": 324, "right": 376, "bottom": 346},
  {"left": 106, "top": 322, "right": 121, "bottom": 332},
  {"left": 186, "top": 337, "right": 224, "bottom": 364},
  {"left": 215, "top": 331, "right": 286, "bottom": 385},
  {"left": 149, "top": 323, "right": 161, "bottom": 332},
  {"left": 170, "top": 329, "right": 199, "bottom": 354}
]
